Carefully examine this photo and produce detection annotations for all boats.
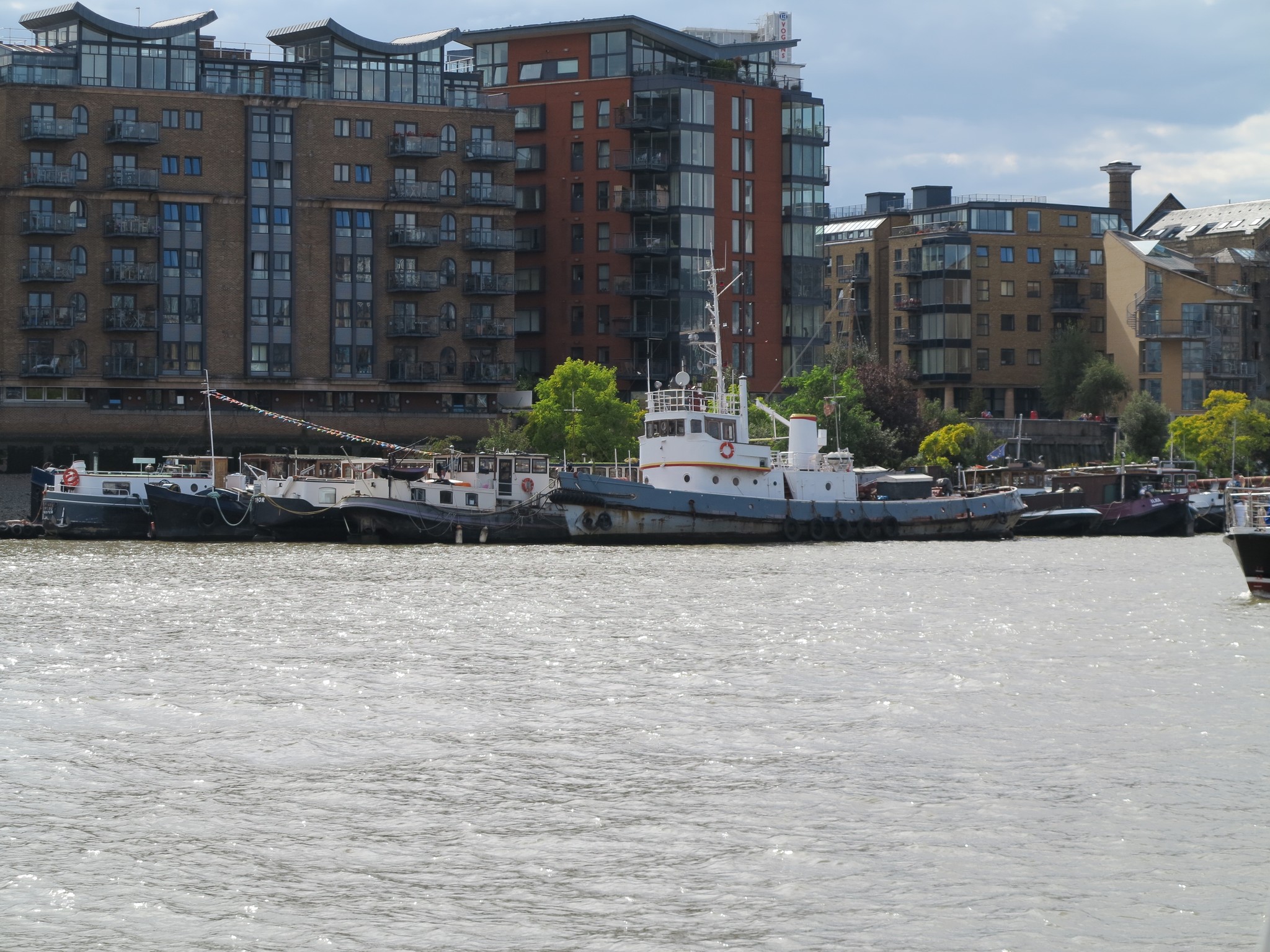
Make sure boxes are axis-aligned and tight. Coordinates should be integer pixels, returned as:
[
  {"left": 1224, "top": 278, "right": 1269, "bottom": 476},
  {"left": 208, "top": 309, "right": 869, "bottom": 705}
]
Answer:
[
  {"left": 42, "top": 241, "right": 1270, "bottom": 541},
  {"left": 1222, "top": 484, "right": 1270, "bottom": 599}
]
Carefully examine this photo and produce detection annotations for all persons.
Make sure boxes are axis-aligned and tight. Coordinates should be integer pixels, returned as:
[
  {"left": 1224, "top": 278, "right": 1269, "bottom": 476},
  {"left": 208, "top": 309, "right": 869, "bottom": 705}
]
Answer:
[
  {"left": 1079, "top": 413, "right": 1094, "bottom": 421},
  {"left": 1138, "top": 484, "right": 1160, "bottom": 498},
  {"left": 1226, "top": 471, "right": 1251, "bottom": 525},
  {"left": 981, "top": 409, "right": 993, "bottom": 418}
]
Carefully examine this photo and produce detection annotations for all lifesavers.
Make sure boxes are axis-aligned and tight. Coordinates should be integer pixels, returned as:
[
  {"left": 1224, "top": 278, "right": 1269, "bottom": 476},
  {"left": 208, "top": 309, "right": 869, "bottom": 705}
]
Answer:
[
  {"left": 64, "top": 473, "right": 79, "bottom": 486},
  {"left": 1188, "top": 482, "right": 1199, "bottom": 493},
  {"left": 720, "top": 442, "right": 734, "bottom": 459},
  {"left": 63, "top": 468, "right": 77, "bottom": 483},
  {"left": 522, "top": 478, "right": 534, "bottom": 492}
]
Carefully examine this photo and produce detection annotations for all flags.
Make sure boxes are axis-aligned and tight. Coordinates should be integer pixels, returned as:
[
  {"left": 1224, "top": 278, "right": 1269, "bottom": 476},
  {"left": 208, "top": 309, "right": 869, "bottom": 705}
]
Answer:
[
  {"left": 202, "top": 391, "right": 443, "bottom": 456},
  {"left": 987, "top": 442, "right": 1006, "bottom": 461}
]
